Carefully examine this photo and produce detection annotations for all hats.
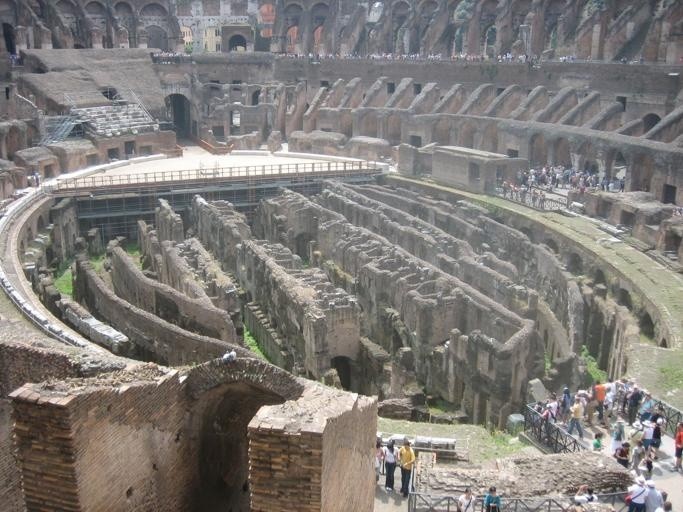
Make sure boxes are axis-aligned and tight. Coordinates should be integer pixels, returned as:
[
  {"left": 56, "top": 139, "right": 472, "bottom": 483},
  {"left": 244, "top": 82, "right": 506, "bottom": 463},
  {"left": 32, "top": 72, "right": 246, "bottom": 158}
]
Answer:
[
  {"left": 636, "top": 476, "right": 646, "bottom": 486},
  {"left": 647, "top": 480, "right": 655, "bottom": 488},
  {"left": 642, "top": 420, "right": 651, "bottom": 427}
]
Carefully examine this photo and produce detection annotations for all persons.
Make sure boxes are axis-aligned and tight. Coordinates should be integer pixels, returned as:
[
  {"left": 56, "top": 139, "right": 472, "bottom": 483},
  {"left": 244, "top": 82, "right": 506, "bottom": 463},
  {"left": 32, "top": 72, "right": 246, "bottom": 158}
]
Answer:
[
  {"left": 160, "top": 50, "right": 180, "bottom": 64},
  {"left": 457, "top": 487, "right": 475, "bottom": 512},
  {"left": 639, "top": 54, "right": 644, "bottom": 63},
  {"left": 483, "top": 486, "right": 500, "bottom": 512},
  {"left": 375, "top": 437, "right": 384, "bottom": 485},
  {"left": 502, "top": 164, "right": 628, "bottom": 209},
  {"left": 531, "top": 377, "right": 683, "bottom": 512},
  {"left": 384, "top": 439, "right": 399, "bottom": 491},
  {"left": 397, "top": 438, "right": 416, "bottom": 497},
  {"left": 622, "top": 56, "right": 628, "bottom": 63}
]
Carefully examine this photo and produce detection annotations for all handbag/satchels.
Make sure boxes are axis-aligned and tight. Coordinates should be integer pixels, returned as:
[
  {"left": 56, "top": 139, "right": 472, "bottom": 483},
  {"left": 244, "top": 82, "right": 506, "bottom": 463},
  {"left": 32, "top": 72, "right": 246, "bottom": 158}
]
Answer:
[{"left": 625, "top": 496, "right": 632, "bottom": 505}]
